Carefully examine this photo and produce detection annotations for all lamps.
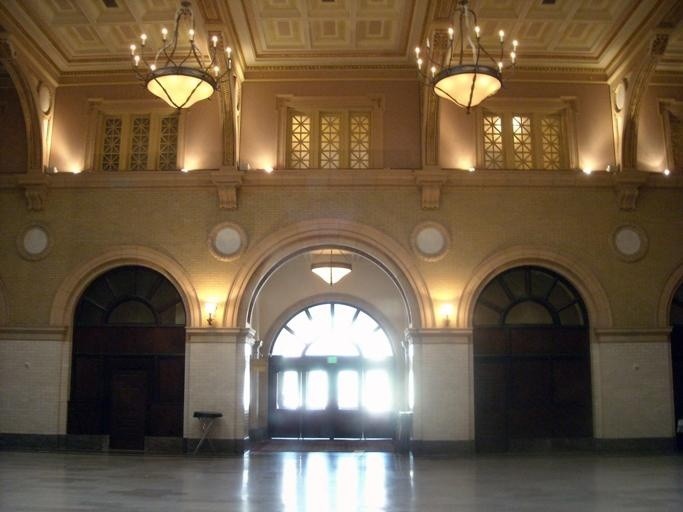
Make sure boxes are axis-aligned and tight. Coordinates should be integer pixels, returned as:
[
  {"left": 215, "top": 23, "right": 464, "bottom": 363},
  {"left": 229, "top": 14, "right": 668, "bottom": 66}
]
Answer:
[
  {"left": 411, "top": 1, "right": 524, "bottom": 113},
  {"left": 126, "top": 1, "right": 235, "bottom": 109},
  {"left": 201, "top": 300, "right": 220, "bottom": 327},
  {"left": 438, "top": 303, "right": 455, "bottom": 326}
]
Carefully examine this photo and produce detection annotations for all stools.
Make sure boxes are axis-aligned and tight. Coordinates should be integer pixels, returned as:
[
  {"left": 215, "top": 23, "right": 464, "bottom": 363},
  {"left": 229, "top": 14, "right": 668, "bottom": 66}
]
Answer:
[{"left": 188, "top": 409, "right": 223, "bottom": 458}]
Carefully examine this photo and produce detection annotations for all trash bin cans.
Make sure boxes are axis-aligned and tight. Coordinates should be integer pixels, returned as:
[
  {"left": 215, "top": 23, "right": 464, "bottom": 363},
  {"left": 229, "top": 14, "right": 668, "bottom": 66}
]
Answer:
[{"left": 393, "top": 412, "right": 411, "bottom": 454}]
